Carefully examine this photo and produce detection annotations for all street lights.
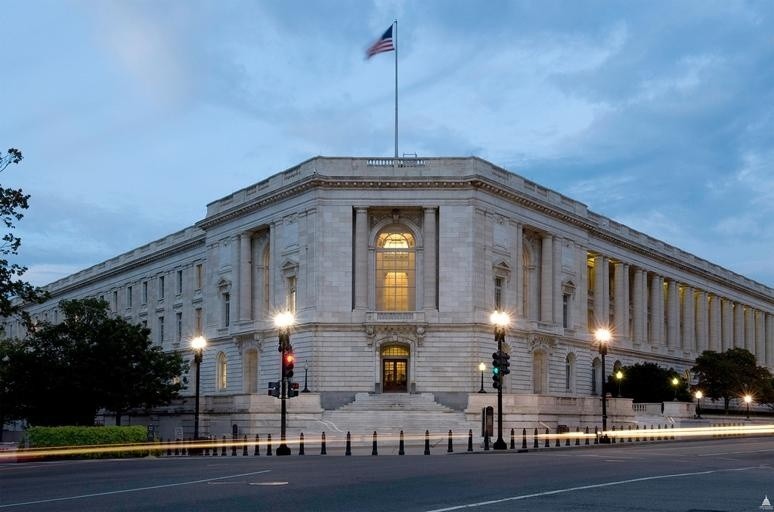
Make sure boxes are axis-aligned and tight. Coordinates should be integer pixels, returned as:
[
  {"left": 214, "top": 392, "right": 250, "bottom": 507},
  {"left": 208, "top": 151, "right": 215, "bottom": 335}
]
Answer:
[
  {"left": 745, "top": 394, "right": 752, "bottom": 420},
  {"left": 478, "top": 361, "right": 485, "bottom": 392},
  {"left": 490, "top": 308, "right": 510, "bottom": 449},
  {"left": 672, "top": 378, "right": 679, "bottom": 401},
  {"left": 301, "top": 360, "right": 310, "bottom": 392},
  {"left": 695, "top": 391, "right": 703, "bottom": 419},
  {"left": 593, "top": 327, "right": 611, "bottom": 444},
  {"left": 191, "top": 336, "right": 206, "bottom": 440},
  {"left": 275, "top": 311, "right": 294, "bottom": 456},
  {"left": 615, "top": 370, "right": 623, "bottom": 397}
]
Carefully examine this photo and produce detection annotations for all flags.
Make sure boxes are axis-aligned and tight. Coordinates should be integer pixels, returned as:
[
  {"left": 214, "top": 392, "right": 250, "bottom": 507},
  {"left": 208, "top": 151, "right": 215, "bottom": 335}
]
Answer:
[{"left": 365, "top": 24, "right": 393, "bottom": 60}]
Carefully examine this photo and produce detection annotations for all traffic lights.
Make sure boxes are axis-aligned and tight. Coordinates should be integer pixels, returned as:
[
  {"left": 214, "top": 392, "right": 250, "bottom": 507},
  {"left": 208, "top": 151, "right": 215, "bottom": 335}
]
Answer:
[
  {"left": 283, "top": 352, "right": 294, "bottom": 377},
  {"left": 492, "top": 351, "right": 500, "bottom": 388},
  {"left": 288, "top": 380, "right": 298, "bottom": 397},
  {"left": 502, "top": 350, "right": 510, "bottom": 375},
  {"left": 267, "top": 380, "right": 280, "bottom": 397}
]
